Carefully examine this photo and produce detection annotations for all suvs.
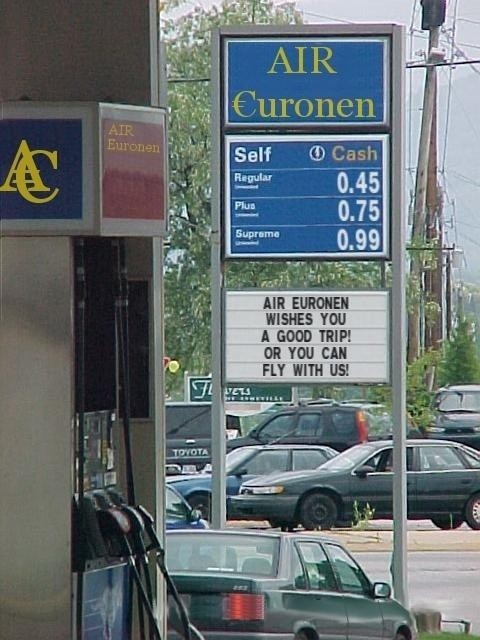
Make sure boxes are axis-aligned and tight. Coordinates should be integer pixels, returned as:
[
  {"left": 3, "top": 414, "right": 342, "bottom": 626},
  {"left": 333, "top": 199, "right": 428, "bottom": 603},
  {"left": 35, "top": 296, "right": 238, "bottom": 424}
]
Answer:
[
  {"left": 224, "top": 398, "right": 423, "bottom": 444},
  {"left": 165, "top": 401, "right": 242, "bottom": 465},
  {"left": 430, "top": 384, "right": 480, "bottom": 449}
]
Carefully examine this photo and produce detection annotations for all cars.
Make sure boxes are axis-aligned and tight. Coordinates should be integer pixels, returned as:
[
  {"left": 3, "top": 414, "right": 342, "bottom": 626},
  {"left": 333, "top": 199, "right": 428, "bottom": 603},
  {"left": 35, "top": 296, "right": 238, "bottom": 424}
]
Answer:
[
  {"left": 166, "top": 482, "right": 209, "bottom": 531},
  {"left": 166, "top": 445, "right": 355, "bottom": 520},
  {"left": 163, "top": 529, "right": 418, "bottom": 639},
  {"left": 228, "top": 438, "right": 479, "bottom": 530}
]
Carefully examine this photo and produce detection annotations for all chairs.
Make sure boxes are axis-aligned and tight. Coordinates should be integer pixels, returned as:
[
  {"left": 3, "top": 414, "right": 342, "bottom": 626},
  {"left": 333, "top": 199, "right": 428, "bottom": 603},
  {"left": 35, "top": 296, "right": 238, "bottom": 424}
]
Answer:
[
  {"left": 187, "top": 555, "right": 208, "bottom": 570},
  {"left": 241, "top": 558, "right": 271, "bottom": 575}
]
